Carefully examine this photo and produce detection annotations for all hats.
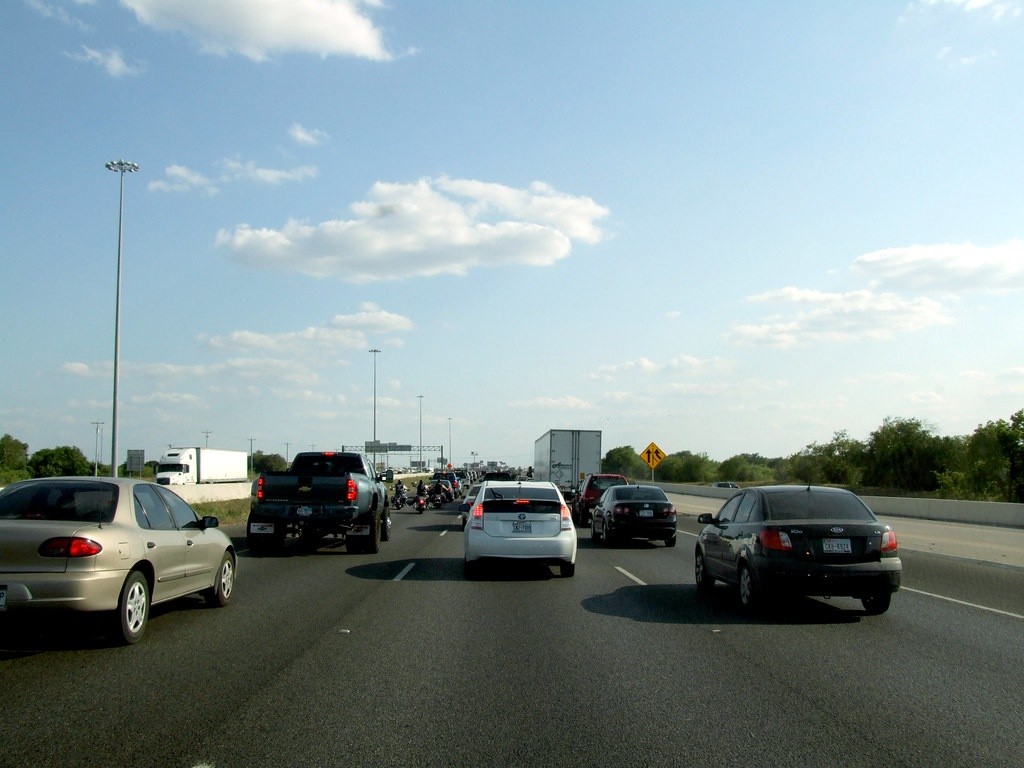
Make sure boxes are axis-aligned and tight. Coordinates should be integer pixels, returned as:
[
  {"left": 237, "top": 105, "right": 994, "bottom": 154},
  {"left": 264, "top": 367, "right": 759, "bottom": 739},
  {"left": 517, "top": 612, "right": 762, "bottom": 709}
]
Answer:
[
  {"left": 419, "top": 479, "right": 424, "bottom": 483},
  {"left": 438, "top": 479, "right": 441, "bottom": 483},
  {"left": 398, "top": 479, "right": 402, "bottom": 483}
]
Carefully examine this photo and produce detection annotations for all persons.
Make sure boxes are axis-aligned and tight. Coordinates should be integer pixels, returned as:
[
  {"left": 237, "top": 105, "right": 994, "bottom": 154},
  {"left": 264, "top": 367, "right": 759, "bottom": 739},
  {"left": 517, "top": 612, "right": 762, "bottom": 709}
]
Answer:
[
  {"left": 430, "top": 479, "right": 449, "bottom": 505},
  {"left": 407, "top": 479, "right": 430, "bottom": 506},
  {"left": 392, "top": 479, "right": 409, "bottom": 507}
]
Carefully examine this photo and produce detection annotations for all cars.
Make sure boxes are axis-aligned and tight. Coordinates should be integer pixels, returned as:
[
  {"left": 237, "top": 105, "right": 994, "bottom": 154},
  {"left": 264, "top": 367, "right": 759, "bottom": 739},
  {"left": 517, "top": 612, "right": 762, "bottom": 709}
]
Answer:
[
  {"left": 459, "top": 483, "right": 482, "bottom": 530},
  {"left": 0, "top": 476, "right": 238, "bottom": 648},
  {"left": 429, "top": 467, "right": 535, "bottom": 498},
  {"left": 589, "top": 485, "right": 677, "bottom": 547},
  {"left": 711, "top": 481, "right": 741, "bottom": 489},
  {"left": 693, "top": 484, "right": 903, "bottom": 613},
  {"left": 457, "top": 481, "right": 578, "bottom": 577}
]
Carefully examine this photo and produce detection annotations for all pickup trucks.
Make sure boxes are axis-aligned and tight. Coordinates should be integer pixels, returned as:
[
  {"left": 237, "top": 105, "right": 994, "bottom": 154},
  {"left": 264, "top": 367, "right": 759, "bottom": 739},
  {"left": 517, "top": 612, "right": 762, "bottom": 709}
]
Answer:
[{"left": 245, "top": 451, "right": 392, "bottom": 554}]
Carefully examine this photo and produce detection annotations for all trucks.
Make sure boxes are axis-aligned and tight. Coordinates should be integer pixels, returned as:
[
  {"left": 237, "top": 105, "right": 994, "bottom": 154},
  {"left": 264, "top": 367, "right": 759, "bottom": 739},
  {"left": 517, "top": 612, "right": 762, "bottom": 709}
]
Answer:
[
  {"left": 153, "top": 447, "right": 248, "bottom": 486},
  {"left": 531, "top": 428, "right": 602, "bottom": 501}
]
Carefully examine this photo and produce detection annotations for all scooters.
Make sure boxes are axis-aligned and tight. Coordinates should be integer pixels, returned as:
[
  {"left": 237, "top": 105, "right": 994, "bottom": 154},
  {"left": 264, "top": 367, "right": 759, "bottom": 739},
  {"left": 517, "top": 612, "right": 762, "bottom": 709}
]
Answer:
[
  {"left": 431, "top": 494, "right": 445, "bottom": 509},
  {"left": 412, "top": 495, "right": 429, "bottom": 514},
  {"left": 392, "top": 488, "right": 410, "bottom": 509}
]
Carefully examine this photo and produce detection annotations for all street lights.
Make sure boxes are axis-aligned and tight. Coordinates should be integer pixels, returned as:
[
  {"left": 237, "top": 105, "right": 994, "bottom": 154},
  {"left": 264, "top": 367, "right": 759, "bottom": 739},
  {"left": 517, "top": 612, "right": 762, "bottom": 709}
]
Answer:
[
  {"left": 202, "top": 431, "right": 213, "bottom": 447},
  {"left": 416, "top": 396, "right": 425, "bottom": 474},
  {"left": 102, "top": 159, "right": 141, "bottom": 474},
  {"left": 447, "top": 417, "right": 454, "bottom": 464},
  {"left": 283, "top": 442, "right": 294, "bottom": 468},
  {"left": 469, "top": 452, "right": 478, "bottom": 468},
  {"left": 246, "top": 437, "right": 258, "bottom": 482},
  {"left": 91, "top": 421, "right": 106, "bottom": 478},
  {"left": 368, "top": 349, "right": 381, "bottom": 471},
  {"left": 309, "top": 445, "right": 320, "bottom": 453}
]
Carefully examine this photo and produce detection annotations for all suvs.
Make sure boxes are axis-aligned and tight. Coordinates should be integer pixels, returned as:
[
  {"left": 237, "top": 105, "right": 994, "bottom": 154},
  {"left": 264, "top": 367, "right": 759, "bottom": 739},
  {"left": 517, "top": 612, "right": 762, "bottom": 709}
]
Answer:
[{"left": 570, "top": 474, "right": 630, "bottom": 527}]
